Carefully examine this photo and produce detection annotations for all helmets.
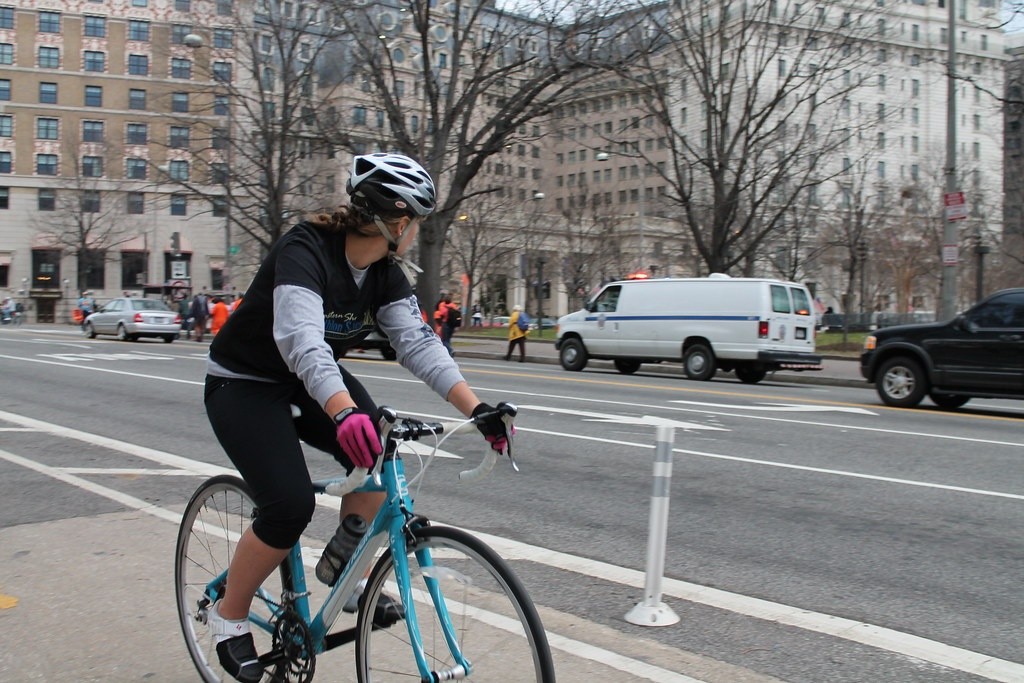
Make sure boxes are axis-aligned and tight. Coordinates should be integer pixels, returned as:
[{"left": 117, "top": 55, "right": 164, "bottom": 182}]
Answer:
[{"left": 346, "top": 152, "right": 436, "bottom": 217}]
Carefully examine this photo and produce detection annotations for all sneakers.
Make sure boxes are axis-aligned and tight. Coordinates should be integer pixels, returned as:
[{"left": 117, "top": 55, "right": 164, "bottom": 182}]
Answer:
[
  {"left": 341, "top": 579, "right": 405, "bottom": 619},
  {"left": 207, "top": 598, "right": 265, "bottom": 683}
]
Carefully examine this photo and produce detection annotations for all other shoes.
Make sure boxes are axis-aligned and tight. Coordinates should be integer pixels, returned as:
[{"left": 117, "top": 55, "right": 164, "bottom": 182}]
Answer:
[
  {"left": 450, "top": 349, "right": 456, "bottom": 357},
  {"left": 4, "top": 317, "right": 11, "bottom": 320}
]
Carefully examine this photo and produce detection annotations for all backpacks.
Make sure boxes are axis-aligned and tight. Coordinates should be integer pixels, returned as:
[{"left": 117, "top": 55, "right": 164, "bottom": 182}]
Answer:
[
  {"left": 513, "top": 312, "right": 530, "bottom": 330},
  {"left": 442, "top": 304, "right": 462, "bottom": 328}
]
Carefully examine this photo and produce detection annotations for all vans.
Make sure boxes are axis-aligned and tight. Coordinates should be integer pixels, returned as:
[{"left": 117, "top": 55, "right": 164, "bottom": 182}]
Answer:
[
  {"left": 554, "top": 272, "right": 824, "bottom": 383},
  {"left": 815, "top": 309, "right": 937, "bottom": 332}
]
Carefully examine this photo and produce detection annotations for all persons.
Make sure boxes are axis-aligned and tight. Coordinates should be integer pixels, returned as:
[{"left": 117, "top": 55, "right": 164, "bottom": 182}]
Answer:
[
  {"left": 502, "top": 303, "right": 528, "bottom": 363},
  {"left": 0, "top": 292, "right": 95, "bottom": 330},
  {"left": 204, "top": 152, "right": 515, "bottom": 683},
  {"left": 422, "top": 292, "right": 483, "bottom": 357},
  {"left": 177, "top": 293, "right": 242, "bottom": 340}
]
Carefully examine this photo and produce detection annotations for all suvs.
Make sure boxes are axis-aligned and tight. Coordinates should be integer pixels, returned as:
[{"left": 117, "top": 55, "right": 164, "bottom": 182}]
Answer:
[{"left": 859, "top": 286, "right": 1024, "bottom": 409}]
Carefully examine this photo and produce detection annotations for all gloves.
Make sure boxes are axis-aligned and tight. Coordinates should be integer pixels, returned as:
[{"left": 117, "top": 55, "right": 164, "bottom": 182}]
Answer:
[
  {"left": 333, "top": 407, "right": 383, "bottom": 468},
  {"left": 470, "top": 402, "right": 516, "bottom": 456}
]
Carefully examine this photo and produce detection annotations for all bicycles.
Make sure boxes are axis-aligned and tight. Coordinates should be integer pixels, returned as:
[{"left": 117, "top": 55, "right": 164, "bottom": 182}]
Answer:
[{"left": 174, "top": 402, "right": 556, "bottom": 683}]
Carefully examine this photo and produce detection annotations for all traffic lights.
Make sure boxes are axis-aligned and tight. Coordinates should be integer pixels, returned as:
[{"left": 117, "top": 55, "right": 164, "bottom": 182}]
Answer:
[{"left": 170, "top": 232, "right": 181, "bottom": 257}]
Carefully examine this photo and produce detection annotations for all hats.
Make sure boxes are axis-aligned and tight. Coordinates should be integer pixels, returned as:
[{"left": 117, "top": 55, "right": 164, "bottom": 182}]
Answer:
[{"left": 513, "top": 305, "right": 523, "bottom": 311}]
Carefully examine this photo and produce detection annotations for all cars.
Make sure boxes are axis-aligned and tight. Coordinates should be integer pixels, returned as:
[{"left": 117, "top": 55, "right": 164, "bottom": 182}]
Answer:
[
  {"left": 473, "top": 316, "right": 555, "bottom": 329},
  {"left": 357, "top": 330, "right": 396, "bottom": 360},
  {"left": 82, "top": 295, "right": 183, "bottom": 344}
]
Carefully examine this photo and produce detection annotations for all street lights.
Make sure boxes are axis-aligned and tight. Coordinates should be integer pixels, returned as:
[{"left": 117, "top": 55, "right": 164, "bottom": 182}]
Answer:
[
  {"left": 516, "top": 193, "right": 546, "bottom": 304},
  {"left": 859, "top": 241, "right": 867, "bottom": 328},
  {"left": 183, "top": 34, "right": 231, "bottom": 293},
  {"left": 597, "top": 151, "right": 643, "bottom": 272},
  {"left": 973, "top": 246, "right": 990, "bottom": 307}
]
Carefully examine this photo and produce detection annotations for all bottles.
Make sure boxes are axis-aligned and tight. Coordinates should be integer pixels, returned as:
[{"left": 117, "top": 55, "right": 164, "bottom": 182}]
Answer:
[{"left": 315, "top": 513, "right": 368, "bottom": 585}]
